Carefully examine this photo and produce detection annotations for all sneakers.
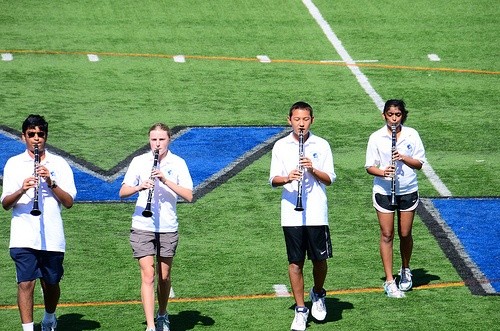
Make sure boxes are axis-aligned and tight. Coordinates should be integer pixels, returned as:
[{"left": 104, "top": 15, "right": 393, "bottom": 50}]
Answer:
[
  {"left": 156, "top": 310, "right": 172, "bottom": 331},
  {"left": 382, "top": 277, "right": 405, "bottom": 298},
  {"left": 399, "top": 268, "right": 413, "bottom": 291},
  {"left": 290, "top": 305, "right": 309, "bottom": 331},
  {"left": 309, "top": 287, "right": 327, "bottom": 321}
]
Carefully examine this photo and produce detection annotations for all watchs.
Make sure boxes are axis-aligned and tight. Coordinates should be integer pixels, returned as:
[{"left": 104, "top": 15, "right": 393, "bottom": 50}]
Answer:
[{"left": 47, "top": 180, "right": 57, "bottom": 190}]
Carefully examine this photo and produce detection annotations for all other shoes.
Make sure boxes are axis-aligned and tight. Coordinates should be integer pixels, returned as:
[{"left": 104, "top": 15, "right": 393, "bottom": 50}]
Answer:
[{"left": 41, "top": 314, "right": 57, "bottom": 331}]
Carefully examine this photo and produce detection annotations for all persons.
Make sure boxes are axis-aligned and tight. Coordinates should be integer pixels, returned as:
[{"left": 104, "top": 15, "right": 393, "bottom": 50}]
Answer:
[
  {"left": 269, "top": 101, "right": 337, "bottom": 331},
  {"left": 1, "top": 114, "right": 77, "bottom": 331},
  {"left": 364, "top": 99, "right": 426, "bottom": 299},
  {"left": 119, "top": 123, "right": 193, "bottom": 331}
]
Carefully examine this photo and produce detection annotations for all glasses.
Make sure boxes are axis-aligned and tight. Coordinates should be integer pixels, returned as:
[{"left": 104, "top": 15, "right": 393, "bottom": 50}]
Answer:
[{"left": 23, "top": 131, "right": 45, "bottom": 137}]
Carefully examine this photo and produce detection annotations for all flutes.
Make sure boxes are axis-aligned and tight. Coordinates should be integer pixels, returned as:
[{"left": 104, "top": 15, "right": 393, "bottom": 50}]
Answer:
[
  {"left": 293, "top": 129, "right": 306, "bottom": 212},
  {"left": 29, "top": 143, "right": 42, "bottom": 216},
  {"left": 389, "top": 123, "right": 398, "bottom": 208},
  {"left": 141, "top": 149, "right": 160, "bottom": 218}
]
